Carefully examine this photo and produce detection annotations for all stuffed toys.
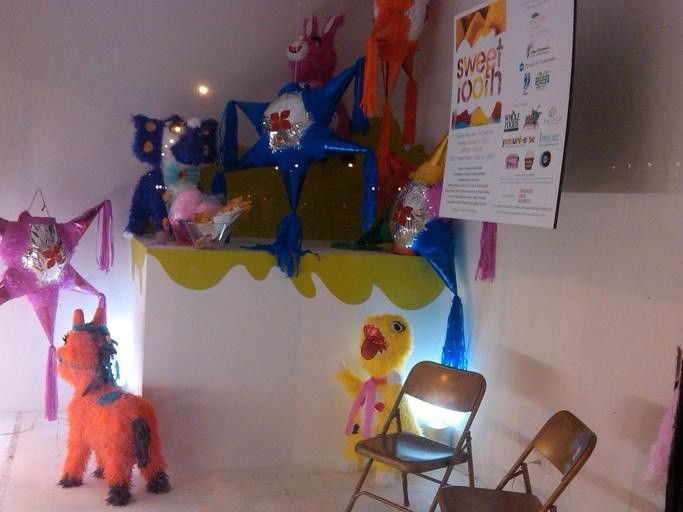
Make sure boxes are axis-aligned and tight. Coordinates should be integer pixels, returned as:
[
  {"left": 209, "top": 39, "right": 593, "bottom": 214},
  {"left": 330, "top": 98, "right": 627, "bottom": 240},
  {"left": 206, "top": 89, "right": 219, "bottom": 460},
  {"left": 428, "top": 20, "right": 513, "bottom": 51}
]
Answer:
[
  {"left": 335, "top": 314, "right": 423, "bottom": 474},
  {"left": 286, "top": 13, "right": 347, "bottom": 144},
  {"left": 120, "top": 112, "right": 220, "bottom": 242},
  {"left": 54, "top": 307, "right": 172, "bottom": 508}
]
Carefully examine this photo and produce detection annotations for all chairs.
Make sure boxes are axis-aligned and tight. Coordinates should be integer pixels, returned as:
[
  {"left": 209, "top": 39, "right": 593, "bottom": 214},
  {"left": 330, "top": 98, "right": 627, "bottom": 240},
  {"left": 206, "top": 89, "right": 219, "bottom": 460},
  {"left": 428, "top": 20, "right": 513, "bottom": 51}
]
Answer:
[
  {"left": 430, "top": 407, "right": 598, "bottom": 511},
  {"left": 344, "top": 358, "right": 487, "bottom": 511}
]
